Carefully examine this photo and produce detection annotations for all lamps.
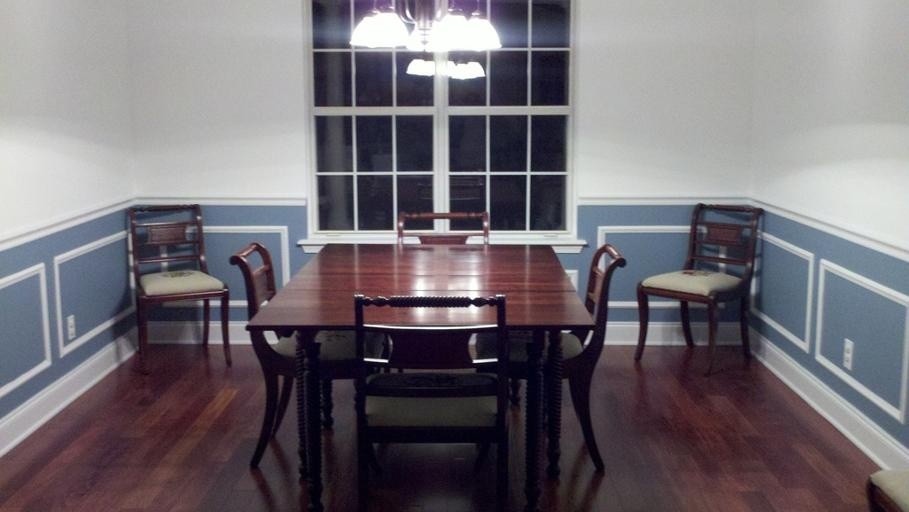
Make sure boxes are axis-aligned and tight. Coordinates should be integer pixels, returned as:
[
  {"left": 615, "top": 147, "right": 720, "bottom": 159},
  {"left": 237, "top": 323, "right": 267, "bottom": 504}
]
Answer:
[{"left": 349, "top": 0, "right": 501, "bottom": 80}]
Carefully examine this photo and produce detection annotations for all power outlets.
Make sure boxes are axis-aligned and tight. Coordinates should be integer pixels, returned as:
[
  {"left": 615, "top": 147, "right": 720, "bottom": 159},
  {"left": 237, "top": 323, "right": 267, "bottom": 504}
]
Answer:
[
  {"left": 68, "top": 316, "right": 77, "bottom": 339},
  {"left": 842, "top": 339, "right": 854, "bottom": 372}
]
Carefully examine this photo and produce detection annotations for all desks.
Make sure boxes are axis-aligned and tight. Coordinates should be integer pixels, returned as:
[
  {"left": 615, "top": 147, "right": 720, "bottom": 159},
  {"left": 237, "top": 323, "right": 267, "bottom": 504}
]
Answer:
[{"left": 245, "top": 243, "right": 596, "bottom": 499}]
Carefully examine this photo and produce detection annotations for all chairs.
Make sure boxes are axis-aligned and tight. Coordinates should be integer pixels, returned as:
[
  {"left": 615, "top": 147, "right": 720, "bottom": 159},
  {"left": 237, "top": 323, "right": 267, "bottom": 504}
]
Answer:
[
  {"left": 126, "top": 203, "right": 233, "bottom": 375},
  {"left": 353, "top": 294, "right": 518, "bottom": 512},
  {"left": 865, "top": 469, "right": 909, "bottom": 512},
  {"left": 229, "top": 241, "right": 384, "bottom": 470},
  {"left": 397, "top": 209, "right": 491, "bottom": 246},
  {"left": 634, "top": 202, "right": 764, "bottom": 377},
  {"left": 467, "top": 245, "right": 627, "bottom": 494}
]
